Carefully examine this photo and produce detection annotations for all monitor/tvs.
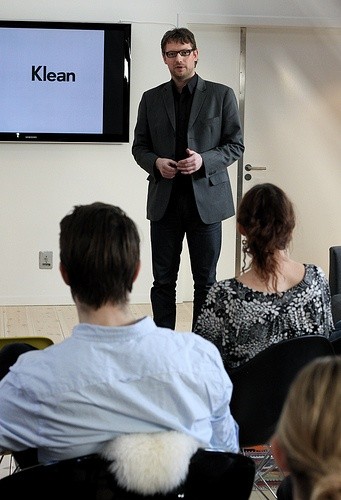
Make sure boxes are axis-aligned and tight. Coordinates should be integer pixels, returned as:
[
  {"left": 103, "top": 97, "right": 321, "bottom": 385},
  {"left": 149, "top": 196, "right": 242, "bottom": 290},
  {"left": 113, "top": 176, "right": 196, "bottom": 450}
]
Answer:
[{"left": 0, "top": 20, "right": 132, "bottom": 144}]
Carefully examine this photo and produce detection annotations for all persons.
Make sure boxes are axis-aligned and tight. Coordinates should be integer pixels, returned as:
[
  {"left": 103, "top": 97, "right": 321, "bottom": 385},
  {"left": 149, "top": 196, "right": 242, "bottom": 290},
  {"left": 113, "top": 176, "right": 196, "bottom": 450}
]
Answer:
[
  {"left": 270, "top": 359, "right": 339, "bottom": 499},
  {"left": 0, "top": 202, "right": 238, "bottom": 467},
  {"left": 193, "top": 184, "right": 332, "bottom": 369},
  {"left": 132, "top": 29, "right": 244, "bottom": 329}
]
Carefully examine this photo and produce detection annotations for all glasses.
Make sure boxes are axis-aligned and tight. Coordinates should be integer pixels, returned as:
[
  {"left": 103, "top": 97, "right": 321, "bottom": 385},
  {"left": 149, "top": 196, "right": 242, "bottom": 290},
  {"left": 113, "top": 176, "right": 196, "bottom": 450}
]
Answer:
[{"left": 164, "top": 48, "right": 195, "bottom": 58}]
